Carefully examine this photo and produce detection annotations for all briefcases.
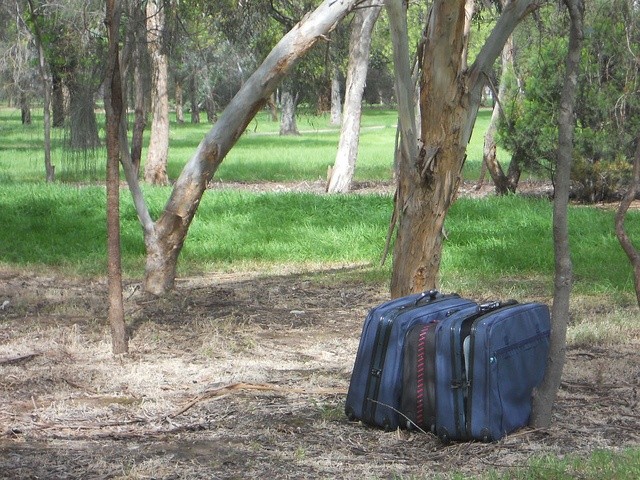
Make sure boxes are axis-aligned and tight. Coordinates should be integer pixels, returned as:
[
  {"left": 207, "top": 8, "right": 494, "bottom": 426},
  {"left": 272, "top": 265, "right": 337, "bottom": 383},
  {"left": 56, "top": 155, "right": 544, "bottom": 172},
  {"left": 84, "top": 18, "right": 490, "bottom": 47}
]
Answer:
[
  {"left": 344, "top": 289, "right": 478, "bottom": 433},
  {"left": 399, "top": 318, "right": 440, "bottom": 432},
  {"left": 434, "top": 299, "right": 550, "bottom": 444}
]
